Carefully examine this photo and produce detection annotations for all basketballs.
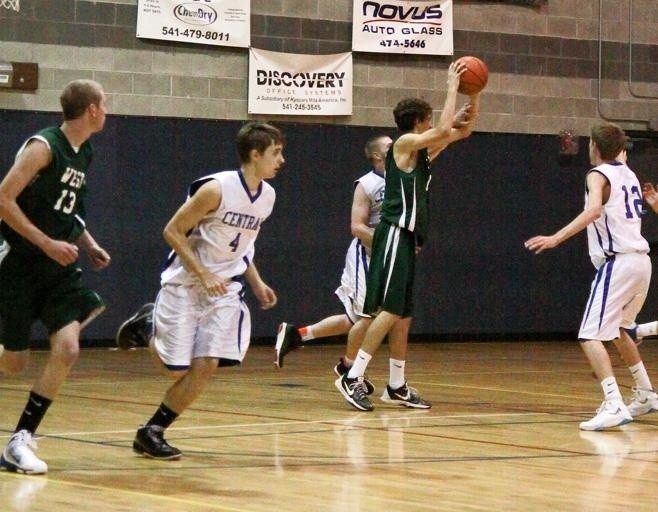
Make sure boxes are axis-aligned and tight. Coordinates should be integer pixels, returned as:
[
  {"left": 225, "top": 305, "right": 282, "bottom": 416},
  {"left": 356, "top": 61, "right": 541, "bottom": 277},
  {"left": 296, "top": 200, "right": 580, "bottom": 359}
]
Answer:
[{"left": 454, "top": 55, "right": 489, "bottom": 96}]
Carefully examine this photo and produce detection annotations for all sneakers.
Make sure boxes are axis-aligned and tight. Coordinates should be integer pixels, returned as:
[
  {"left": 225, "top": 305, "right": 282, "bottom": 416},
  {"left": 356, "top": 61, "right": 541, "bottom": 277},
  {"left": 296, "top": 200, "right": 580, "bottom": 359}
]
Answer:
[
  {"left": 117, "top": 303, "right": 155, "bottom": 350},
  {"left": 627, "top": 390, "right": 658, "bottom": 417},
  {"left": 580, "top": 400, "right": 633, "bottom": 432},
  {"left": 334, "top": 358, "right": 376, "bottom": 411},
  {"left": 380, "top": 382, "right": 432, "bottom": 408},
  {"left": 132, "top": 425, "right": 183, "bottom": 460},
  {"left": 1, "top": 429, "right": 48, "bottom": 475},
  {"left": 619, "top": 323, "right": 637, "bottom": 360},
  {"left": 274, "top": 322, "right": 302, "bottom": 368}
]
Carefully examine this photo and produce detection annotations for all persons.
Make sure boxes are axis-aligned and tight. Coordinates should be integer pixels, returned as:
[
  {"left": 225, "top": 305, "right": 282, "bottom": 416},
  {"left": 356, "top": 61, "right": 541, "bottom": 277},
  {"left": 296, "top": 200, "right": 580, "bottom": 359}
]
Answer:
[
  {"left": 619, "top": 317, "right": 658, "bottom": 361},
  {"left": 114, "top": 119, "right": 287, "bottom": 462},
  {"left": 272, "top": 133, "right": 395, "bottom": 396},
  {"left": 333, "top": 412, "right": 412, "bottom": 512},
  {"left": 1, "top": 78, "right": 111, "bottom": 477},
  {"left": 523, "top": 122, "right": 658, "bottom": 432},
  {"left": 332, "top": 58, "right": 481, "bottom": 412},
  {"left": 642, "top": 178, "right": 658, "bottom": 217},
  {"left": 575, "top": 424, "right": 658, "bottom": 511}
]
